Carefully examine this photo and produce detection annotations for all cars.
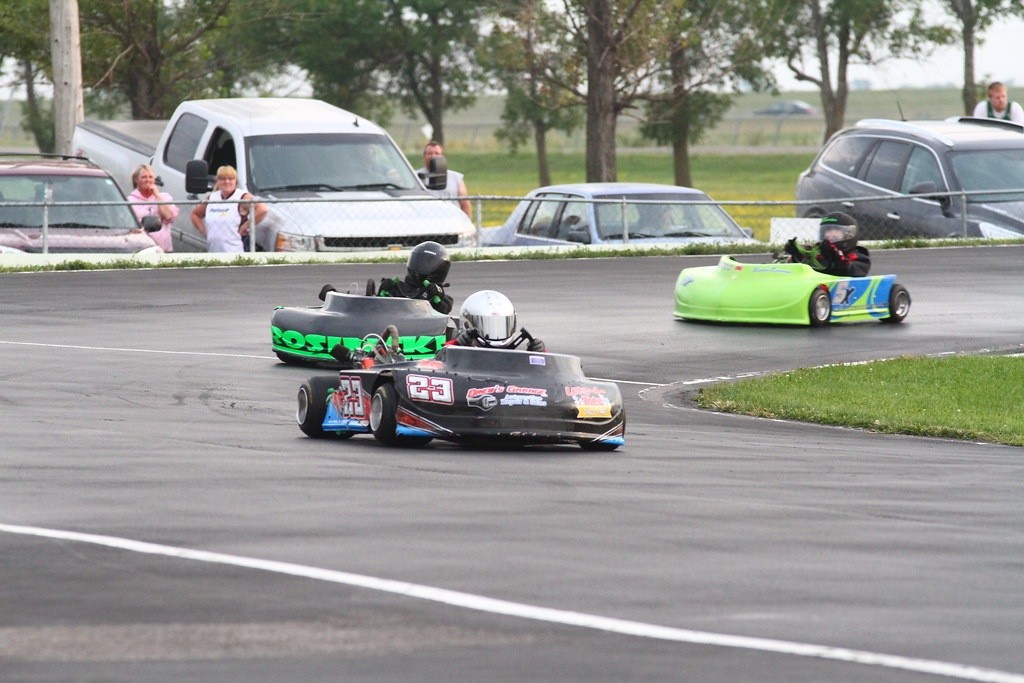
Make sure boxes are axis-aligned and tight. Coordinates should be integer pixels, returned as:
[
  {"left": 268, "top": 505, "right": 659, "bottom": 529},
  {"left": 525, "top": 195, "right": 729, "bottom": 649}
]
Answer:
[{"left": 473, "top": 181, "right": 763, "bottom": 247}]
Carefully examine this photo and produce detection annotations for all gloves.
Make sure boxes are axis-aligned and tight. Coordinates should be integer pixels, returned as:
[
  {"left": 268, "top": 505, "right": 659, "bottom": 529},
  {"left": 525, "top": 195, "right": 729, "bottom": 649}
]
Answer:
[
  {"left": 456, "top": 327, "right": 480, "bottom": 346},
  {"left": 378, "top": 277, "right": 395, "bottom": 297},
  {"left": 426, "top": 283, "right": 445, "bottom": 300},
  {"left": 784, "top": 237, "right": 803, "bottom": 257},
  {"left": 818, "top": 238, "right": 845, "bottom": 264},
  {"left": 526, "top": 338, "right": 545, "bottom": 352}
]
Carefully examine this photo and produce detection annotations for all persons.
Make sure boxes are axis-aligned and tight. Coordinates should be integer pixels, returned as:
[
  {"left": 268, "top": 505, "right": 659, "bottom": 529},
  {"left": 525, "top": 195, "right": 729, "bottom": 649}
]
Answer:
[
  {"left": 425, "top": 287, "right": 550, "bottom": 369},
  {"left": 412, "top": 140, "right": 473, "bottom": 224},
  {"left": 970, "top": 77, "right": 1023, "bottom": 129},
  {"left": 777, "top": 209, "right": 875, "bottom": 279},
  {"left": 364, "top": 238, "right": 459, "bottom": 320},
  {"left": 127, "top": 164, "right": 183, "bottom": 252},
  {"left": 187, "top": 165, "right": 270, "bottom": 254}
]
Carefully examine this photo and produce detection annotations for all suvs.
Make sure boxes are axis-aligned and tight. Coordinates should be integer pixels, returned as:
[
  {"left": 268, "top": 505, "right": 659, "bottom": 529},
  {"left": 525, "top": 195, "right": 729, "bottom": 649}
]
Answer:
[
  {"left": 1, "top": 150, "right": 167, "bottom": 268},
  {"left": 795, "top": 99, "right": 1024, "bottom": 242}
]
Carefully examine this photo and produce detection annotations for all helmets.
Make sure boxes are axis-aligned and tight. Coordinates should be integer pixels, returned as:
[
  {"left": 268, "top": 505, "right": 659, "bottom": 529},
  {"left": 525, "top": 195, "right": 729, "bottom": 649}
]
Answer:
[
  {"left": 820, "top": 211, "right": 859, "bottom": 252},
  {"left": 404, "top": 241, "right": 452, "bottom": 289},
  {"left": 459, "top": 290, "right": 518, "bottom": 348}
]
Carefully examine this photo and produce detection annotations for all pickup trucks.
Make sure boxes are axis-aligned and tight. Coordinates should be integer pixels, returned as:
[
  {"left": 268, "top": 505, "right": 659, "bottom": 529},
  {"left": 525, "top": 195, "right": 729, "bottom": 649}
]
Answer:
[{"left": 73, "top": 96, "right": 479, "bottom": 254}]
